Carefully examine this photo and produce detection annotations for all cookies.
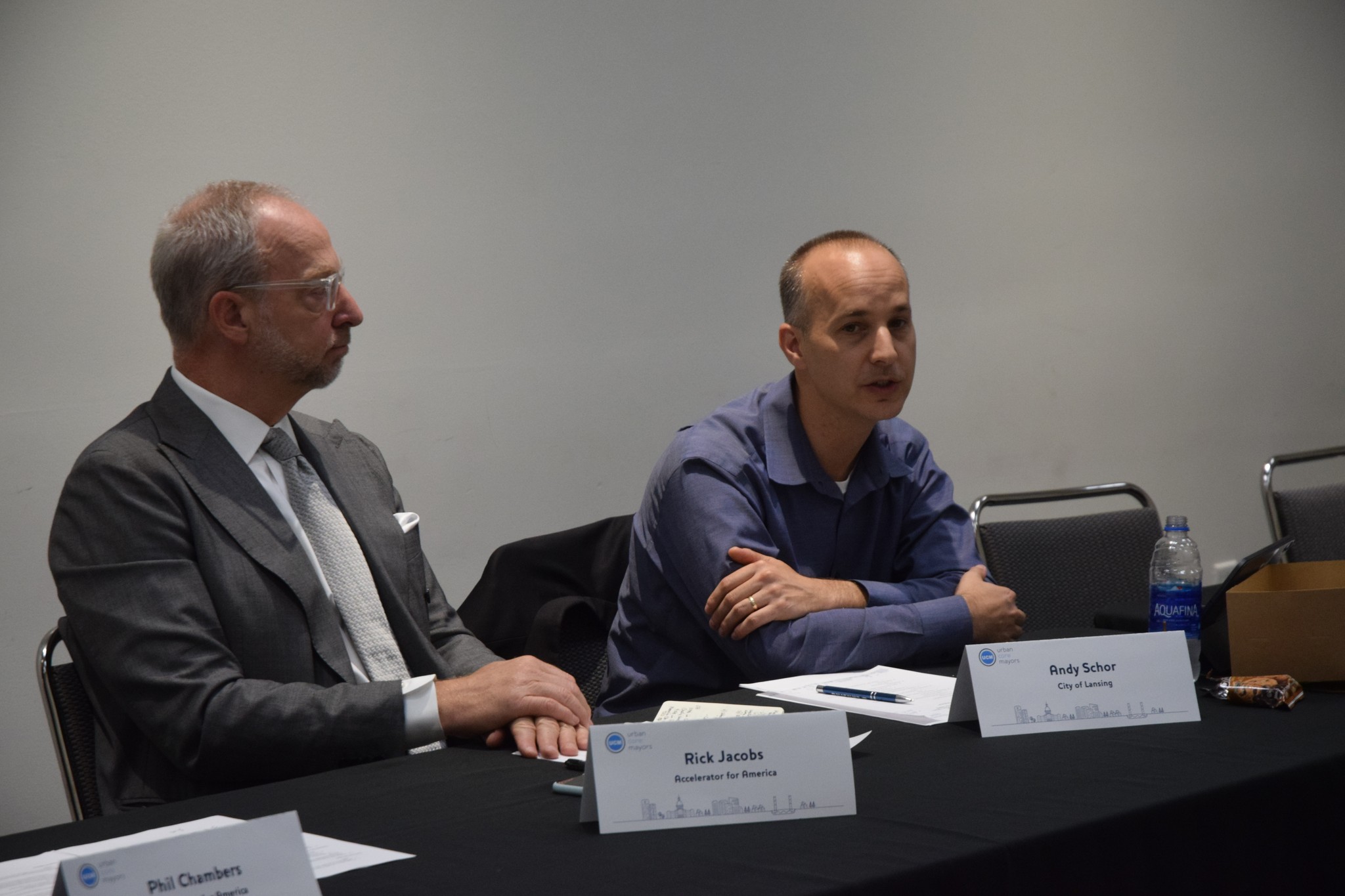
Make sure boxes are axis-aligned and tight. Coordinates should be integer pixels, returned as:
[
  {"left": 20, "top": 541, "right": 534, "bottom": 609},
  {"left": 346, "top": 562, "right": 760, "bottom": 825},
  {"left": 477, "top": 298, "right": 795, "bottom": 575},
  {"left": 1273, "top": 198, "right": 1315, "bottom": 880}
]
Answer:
[{"left": 1236, "top": 675, "right": 1288, "bottom": 699}]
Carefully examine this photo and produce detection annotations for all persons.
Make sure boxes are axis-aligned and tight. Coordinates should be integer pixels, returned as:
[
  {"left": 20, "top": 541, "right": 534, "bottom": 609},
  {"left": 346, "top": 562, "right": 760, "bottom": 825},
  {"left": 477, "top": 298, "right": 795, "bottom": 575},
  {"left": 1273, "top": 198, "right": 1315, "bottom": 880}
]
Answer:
[
  {"left": 595, "top": 230, "right": 1027, "bottom": 719},
  {"left": 47, "top": 179, "right": 599, "bottom": 820}
]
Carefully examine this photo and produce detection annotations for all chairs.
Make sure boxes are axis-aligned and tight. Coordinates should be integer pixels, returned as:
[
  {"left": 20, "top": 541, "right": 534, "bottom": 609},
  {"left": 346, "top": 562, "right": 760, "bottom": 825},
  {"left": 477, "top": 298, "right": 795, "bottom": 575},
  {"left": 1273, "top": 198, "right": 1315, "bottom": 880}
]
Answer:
[
  {"left": 456, "top": 510, "right": 638, "bottom": 721},
  {"left": 1260, "top": 442, "right": 1345, "bottom": 564},
  {"left": 34, "top": 611, "right": 107, "bottom": 822},
  {"left": 966, "top": 479, "right": 1179, "bottom": 634}
]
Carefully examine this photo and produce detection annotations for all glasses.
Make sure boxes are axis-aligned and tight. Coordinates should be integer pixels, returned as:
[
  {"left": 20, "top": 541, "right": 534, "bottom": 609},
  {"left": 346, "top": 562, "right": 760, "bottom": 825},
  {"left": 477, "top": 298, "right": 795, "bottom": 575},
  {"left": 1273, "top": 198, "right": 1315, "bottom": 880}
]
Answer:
[{"left": 230, "top": 266, "right": 345, "bottom": 312}]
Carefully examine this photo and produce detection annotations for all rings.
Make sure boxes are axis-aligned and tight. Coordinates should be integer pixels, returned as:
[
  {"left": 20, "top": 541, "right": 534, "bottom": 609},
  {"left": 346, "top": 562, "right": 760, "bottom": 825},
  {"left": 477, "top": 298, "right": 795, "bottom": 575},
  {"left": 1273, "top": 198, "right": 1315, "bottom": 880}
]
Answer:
[{"left": 748, "top": 596, "right": 758, "bottom": 610}]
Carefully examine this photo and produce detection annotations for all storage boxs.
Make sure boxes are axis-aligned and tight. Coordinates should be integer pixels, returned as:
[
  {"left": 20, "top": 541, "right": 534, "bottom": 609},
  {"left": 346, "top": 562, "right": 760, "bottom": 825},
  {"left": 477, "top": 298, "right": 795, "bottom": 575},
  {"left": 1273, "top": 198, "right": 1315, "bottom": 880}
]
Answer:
[{"left": 1223, "top": 556, "right": 1345, "bottom": 685}]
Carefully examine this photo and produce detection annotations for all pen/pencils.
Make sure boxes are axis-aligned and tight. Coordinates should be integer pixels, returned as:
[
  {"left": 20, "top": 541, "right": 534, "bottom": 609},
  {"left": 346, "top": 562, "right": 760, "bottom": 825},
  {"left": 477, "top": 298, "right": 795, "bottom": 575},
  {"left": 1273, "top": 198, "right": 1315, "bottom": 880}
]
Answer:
[{"left": 816, "top": 685, "right": 915, "bottom": 704}]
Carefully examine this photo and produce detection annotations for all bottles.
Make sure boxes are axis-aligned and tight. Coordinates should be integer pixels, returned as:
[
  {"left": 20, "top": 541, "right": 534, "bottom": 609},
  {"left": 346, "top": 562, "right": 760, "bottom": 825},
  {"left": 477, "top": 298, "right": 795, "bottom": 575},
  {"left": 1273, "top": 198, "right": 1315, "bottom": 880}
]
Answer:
[{"left": 1149, "top": 515, "right": 1202, "bottom": 684}]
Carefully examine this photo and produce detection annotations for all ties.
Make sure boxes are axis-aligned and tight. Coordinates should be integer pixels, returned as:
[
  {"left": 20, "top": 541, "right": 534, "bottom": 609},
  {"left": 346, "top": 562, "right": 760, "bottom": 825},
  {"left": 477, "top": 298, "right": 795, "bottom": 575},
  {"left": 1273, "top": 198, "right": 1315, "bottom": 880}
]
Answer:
[{"left": 259, "top": 425, "right": 442, "bottom": 756}]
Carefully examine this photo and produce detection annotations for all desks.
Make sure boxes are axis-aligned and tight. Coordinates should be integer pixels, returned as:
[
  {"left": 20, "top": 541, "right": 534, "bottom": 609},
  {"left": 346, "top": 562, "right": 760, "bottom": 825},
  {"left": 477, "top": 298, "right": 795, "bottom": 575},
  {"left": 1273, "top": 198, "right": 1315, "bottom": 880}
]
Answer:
[{"left": 0, "top": 625, "right": 1345, "bottom": 896}]
